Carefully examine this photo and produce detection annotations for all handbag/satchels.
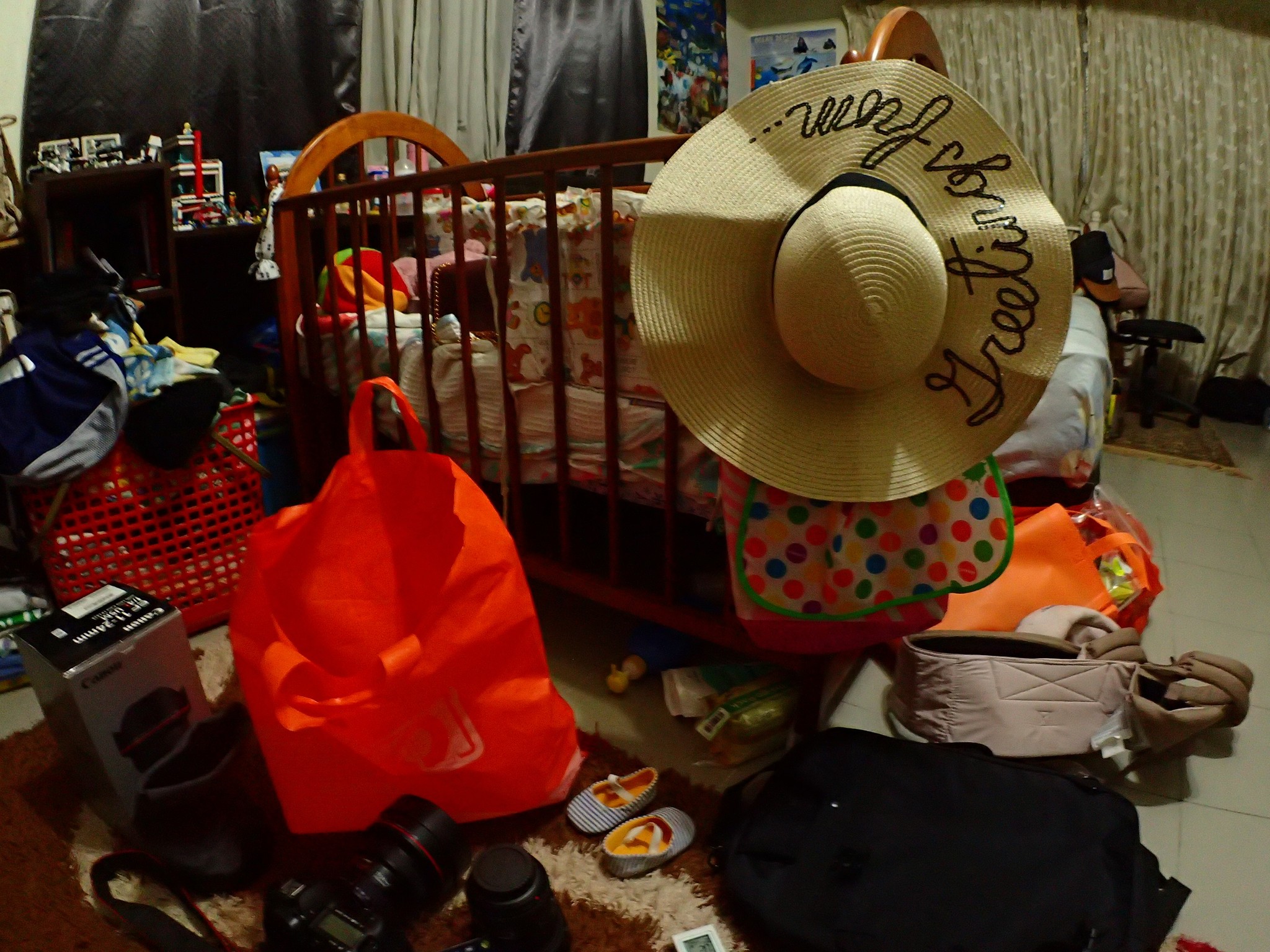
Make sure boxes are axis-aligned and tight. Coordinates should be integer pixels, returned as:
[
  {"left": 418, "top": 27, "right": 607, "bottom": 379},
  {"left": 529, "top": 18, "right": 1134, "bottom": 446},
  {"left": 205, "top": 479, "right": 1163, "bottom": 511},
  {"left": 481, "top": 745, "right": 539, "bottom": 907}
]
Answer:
[
  {"left": 920, "top": 503, "right": 1150, "bottom": 630},
  {"left": 227, "top": 378, "right": 582, "bottom": 836},
  {"left": 1112, "top": 251, "right": 1149, "bottom": 307},
  {"left": 883, "top": 630, "right": 1254, "bottom": 773}
]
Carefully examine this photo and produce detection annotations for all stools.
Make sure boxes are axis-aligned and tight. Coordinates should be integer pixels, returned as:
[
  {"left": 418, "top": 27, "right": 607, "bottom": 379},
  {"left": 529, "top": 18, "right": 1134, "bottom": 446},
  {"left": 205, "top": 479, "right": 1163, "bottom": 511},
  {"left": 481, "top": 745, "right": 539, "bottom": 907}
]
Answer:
[{"left": 1116, "top": 318, "right": 1205, "bottom": 427}]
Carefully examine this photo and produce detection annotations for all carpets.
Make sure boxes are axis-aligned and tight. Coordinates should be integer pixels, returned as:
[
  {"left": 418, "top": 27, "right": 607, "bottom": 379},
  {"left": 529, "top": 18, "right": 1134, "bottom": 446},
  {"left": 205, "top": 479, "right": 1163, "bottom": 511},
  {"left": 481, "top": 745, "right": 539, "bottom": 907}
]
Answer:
[{"left": 1101, "top": 391, "right": 1252, "bottom": 481}]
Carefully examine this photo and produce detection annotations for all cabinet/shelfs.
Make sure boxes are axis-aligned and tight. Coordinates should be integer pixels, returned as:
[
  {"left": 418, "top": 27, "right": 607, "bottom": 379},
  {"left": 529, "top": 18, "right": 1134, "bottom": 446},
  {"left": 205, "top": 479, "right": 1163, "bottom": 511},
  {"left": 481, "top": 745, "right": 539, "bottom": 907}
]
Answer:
[{"left": 22, "top": 157, "right": 417, "bottom": 347}]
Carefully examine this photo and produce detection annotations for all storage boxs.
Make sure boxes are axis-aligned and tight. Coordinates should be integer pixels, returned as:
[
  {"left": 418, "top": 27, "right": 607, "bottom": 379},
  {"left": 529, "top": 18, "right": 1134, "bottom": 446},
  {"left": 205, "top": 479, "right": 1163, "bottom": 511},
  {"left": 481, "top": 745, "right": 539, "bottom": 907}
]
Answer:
[{"left": 11, "top": 583, "right": 208, "bottom": 842}]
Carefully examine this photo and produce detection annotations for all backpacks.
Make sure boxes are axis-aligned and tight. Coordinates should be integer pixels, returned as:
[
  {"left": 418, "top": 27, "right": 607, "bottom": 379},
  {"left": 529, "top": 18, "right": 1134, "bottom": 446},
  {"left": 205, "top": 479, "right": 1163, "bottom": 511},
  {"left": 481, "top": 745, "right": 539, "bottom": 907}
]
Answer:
[{"left": 710, "top": 726, "right": 1190, "bottom": 952}]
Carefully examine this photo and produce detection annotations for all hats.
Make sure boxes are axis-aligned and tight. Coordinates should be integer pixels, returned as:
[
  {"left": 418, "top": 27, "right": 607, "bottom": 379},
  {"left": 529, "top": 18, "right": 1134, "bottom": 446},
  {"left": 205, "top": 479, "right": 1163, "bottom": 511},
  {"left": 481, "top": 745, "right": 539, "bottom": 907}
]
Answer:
[
  {"left": 1070, "top": 231, "right": 1121, "bottom": 302},
  {"left": 631, "top": 58, "right": 1074, "bottom": 503}
]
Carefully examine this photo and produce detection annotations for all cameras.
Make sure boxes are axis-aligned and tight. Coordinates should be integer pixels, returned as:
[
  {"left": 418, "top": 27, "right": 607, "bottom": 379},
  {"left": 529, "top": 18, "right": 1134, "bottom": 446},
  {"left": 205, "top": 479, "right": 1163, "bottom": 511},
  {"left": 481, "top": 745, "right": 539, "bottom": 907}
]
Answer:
[{"left": 264, "top": 795, "right": 472, "bottom": 952}]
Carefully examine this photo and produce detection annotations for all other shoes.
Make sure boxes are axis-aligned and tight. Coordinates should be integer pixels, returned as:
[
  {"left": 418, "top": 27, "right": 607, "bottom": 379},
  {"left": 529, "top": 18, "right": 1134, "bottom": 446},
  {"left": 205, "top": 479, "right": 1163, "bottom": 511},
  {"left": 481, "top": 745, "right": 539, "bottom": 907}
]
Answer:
[{"left": 566, "top": 767, "right": 695, "bottom": 878}]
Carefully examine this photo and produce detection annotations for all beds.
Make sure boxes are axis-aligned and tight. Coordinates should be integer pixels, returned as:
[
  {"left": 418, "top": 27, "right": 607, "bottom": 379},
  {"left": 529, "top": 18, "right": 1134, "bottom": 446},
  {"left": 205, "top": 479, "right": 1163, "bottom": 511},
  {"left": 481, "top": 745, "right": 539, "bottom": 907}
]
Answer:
[{"left": 273, "top": 109, "right": 823, "bottom": 748}]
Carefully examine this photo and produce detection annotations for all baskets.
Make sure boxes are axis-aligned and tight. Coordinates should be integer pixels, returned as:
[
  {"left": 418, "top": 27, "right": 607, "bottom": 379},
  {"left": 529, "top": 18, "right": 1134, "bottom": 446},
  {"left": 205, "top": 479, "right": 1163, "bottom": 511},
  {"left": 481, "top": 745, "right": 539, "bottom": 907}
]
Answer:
[{"left": 17, "top": 394, "right": 263, "bottom": 637}]
